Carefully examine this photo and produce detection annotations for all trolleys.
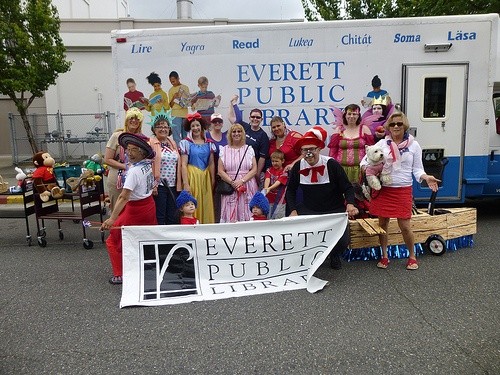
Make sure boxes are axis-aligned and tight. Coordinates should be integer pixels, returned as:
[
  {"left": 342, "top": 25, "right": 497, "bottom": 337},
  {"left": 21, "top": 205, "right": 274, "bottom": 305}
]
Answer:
[{"left": 21, "top": 176, "right": 105, "bottom": 250}]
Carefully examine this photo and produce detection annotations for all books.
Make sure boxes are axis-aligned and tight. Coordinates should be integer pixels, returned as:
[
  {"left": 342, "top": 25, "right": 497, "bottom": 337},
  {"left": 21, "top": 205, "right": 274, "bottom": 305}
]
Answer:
[
  {"left": 173, "top": 87, "right": 197, "bottom": 108},
  {"left": 124, "top": 97, "right": 144, "bottom": 108},
  {"left": 141, "top": 95, "right": 162, "bottom": 107},
  {"left": 196, "top": 95, "right": 219, "bottom": 110}
]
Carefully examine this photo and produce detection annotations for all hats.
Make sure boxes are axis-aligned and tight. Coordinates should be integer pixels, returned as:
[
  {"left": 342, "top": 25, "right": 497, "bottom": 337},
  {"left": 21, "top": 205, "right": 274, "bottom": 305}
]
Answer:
[
  {"left": 249, "top": 191, "right": 270, "bottom": 215},
  {"left": 371, "top": 95, "right": 390, "bottom": 106},
  {"left": 295, "top": 131, "right": 325, "bottom": 153},
  {"left": 176, "top": 190, "right": 197, "bottom": 209},
  {"left": 210, "top": 113, "right": 223, "bottom": 121},
  {"left": 118, "top": 132, "right": 154, "bottom": 159}
]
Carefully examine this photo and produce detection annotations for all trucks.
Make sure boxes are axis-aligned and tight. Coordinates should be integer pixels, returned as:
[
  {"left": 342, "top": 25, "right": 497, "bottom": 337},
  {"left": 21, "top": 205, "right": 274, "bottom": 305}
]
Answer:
[{"left": 110, "top": 13, "right": 500, "bottom": 215}]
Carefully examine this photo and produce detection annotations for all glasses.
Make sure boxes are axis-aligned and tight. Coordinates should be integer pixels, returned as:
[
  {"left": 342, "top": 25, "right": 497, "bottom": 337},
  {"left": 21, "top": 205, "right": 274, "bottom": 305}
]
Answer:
[
  {"left": 251, "top": 116, "right": 262, "bottom": 119},
  {"left": 300, "top": 146, "right": 319, "bottom": 153},
  {"left": 389, "top": 122, "right": 403, "bottom": 127},
  {"left": 155, "top": 126, "right": 170, "bottom": 130},
  {"left": 124, "top": 149, "right": 142, "bottom": 154}
]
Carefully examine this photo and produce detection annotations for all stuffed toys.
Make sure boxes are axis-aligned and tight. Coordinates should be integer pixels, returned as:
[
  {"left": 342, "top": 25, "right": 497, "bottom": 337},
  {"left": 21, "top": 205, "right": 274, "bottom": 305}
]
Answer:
[
  {"left": 364, "top": 145, "right": 392, "bottom": 191},
  {"left": 10, "top": 151, "right": 105, "bottom": 202}
]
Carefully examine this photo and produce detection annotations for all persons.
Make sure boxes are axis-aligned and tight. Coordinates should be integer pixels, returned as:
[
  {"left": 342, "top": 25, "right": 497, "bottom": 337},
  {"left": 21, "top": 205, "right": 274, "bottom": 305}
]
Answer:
[
  {"left": 360, "top": 112, "right": 441, "bottom": 270},
  {"left": 176, "top": 190, "right": 200, "bottom": 225},
  {"left": 205, "top": 113, "right": 227, "bottom": 223},
  {"left": 150, "top": 118, "right": 178, "bottom": 224},
  {"left": 228, "top": 95, "right": 270, "bottom": 186},
  {"left": 264, "top": 149, "right": 287, "bottom": 219},
  {"left": 327, "top": 103, "right": 375, "bottom": 218},
  {"left": 284, "top": 127, "right": 360, "bottom": 270},
  {"left": 249, "top": 192, "right": 269, "bottom": 221},
  {"left": 177, "top": 113, "right": 217, "bottom": 223},
  {"left": 363, "top": 95, "right": 397, "bottom": 144},
  {"left": 99, "top": 133, "right": 158, "bottom": 284},
  {"left": 123, "top": 70, "right": 220, "bottom": 142},
  {"left": 105, "top": 106, "right": 150, "bottom": 218},
  {"left": 361, "top": 75, "right": 386, "bottom": 106},
  {"left": 269, "top": 116, "right": 304, "bottom": 172},
  {"left": 217, "top": 125, "right": 259, "bottom": 223}
]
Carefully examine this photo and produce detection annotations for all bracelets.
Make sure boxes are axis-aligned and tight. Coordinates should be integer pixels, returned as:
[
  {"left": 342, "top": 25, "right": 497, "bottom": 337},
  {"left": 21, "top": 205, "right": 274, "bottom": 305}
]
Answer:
[{"left": 242, "top": 179, "right": 245, "bottom": 183}]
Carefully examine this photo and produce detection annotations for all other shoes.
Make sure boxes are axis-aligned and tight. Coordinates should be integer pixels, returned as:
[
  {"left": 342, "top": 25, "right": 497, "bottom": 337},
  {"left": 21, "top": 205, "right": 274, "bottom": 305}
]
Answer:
[
  {"left": 406, "top": 259, "right": 418, "bottom": 269},
  {"left": 109, "top": 276, "right": 122, "bottom": 284},
  {"left": 329, "top": 254, "right": 342, "bottom": 270},
  {"left": 377, "top": 258, "right": 390, "bottom": 268}
]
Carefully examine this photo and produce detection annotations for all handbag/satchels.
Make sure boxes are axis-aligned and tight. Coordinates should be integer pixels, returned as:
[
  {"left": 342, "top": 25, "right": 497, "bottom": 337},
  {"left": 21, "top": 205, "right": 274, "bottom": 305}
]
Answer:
[{"left": 215, "top": 178, "right": 233, "bottom": 195}]
